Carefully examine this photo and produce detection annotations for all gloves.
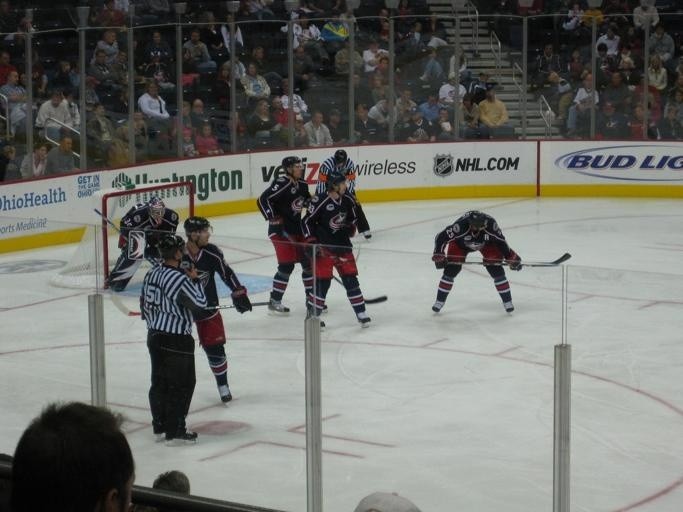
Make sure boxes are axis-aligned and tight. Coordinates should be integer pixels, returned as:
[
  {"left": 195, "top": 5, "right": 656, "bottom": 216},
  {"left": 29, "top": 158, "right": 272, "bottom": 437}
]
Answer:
[
  {"left": 434, "top": 256, "right": 447, "bottom": 268},
  {"left": 232, "top": 286, "right": 252, "bottom": 313},
  {"left": 507, "top": 251, "right": 522, "bottom": 270}
]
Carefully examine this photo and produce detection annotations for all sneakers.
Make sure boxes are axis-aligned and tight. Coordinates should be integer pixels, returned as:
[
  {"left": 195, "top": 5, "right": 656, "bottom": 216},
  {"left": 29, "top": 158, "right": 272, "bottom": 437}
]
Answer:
[
  {"left": 153, "top": 427, "right": 197, "bottom": 440},
  {"left": 219, "top": 384, "right": 232, "bottom": 402},
  {"left": 357, "top": 312, "right": 370, "bottom": 322},
  {"left": 270, "top": 300, "right": 289, "bottom": 312},
  {"left": 306, "top": 315, "right": 325, "bottom": 327},
  {"left": 504, "top": 301, "right": 513, "bottom": 312},
  {"left": 433, "top": 301, "right": 444, "bottom": 312}
]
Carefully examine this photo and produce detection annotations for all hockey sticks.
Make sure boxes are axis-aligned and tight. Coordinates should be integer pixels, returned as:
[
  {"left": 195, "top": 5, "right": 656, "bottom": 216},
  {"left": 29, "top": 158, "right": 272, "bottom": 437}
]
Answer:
[
  {"left": 284, "top": 231, "right": 388, "bottom": 303},
  {"left": 109, "top": 292, "right": 272, "bottom": 316},
  {"left": 93, "top": 208, "right": 157, "bottom": 266},
  {"left": 447, "top": 253, "right": 572, "bottom": 268}
]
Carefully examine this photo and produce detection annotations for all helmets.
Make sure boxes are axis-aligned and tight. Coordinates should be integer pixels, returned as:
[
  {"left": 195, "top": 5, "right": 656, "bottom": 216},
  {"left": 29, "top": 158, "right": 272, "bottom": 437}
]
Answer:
[
  {"left": 327, "top": 172, "right": 344, "bottom": 190},
  {"left": 470, "top": 212, "right": 485, "bottom": 228},
  {"left": 335, "top": 150, "right": 347, "bottom": 164},
  {"left": 282, "top": 156, "right": 299, "bottom": 171},
  {"left": 148, "top": 197, "right": 165, "bottom": 219},
  {"left": 160, "top": 236, "right": 184, "bottom": 259},
  {"left": 185, "top": 216, "right": 210, "bottom": 236}
]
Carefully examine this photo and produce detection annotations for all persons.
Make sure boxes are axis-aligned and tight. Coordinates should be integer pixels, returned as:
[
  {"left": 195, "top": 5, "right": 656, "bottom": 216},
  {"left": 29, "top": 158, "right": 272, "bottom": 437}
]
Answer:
[
  {"left": 138, "top": 232, "right": 206, "bottom": 443},
  {"left": 302, "top": 174, "right": 377, "bottom": 330},
  {"left": 0, "top": 1, "right": 681, "bottom": 180},
  {"left": 350, "top": 490, "right": 422, "bottom": 512},
  {"left": 150, "top": 468, "right": 192, "bottom": 499},
  {"left": 176, "top": 216, "right": 254, "bottom": 404},
  {"left": 314, "top": 147, "right": 374, "bottom": 244},
  {"left": 252, "top": 154, "right": 329, "bottom": 315},
  {"left": 427, "top": 207, "right": 523, "bottom": 313},
  {"left": 100, "top": 192, "right": 183, "bottom": 289},
  {"left": 6, "top": 398, "right": 132, "bottom": 512}
]
